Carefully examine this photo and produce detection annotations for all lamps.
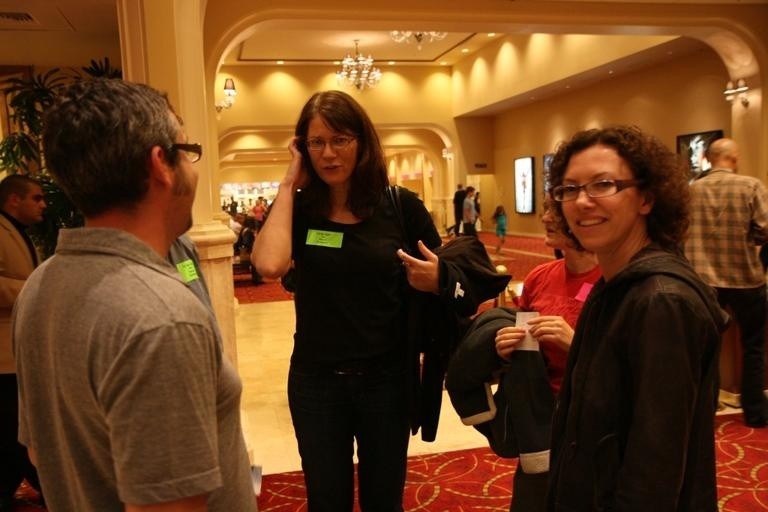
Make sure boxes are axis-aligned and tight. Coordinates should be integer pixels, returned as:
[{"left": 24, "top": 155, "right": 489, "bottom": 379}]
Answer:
[
  {"left": 388, "top": 30, "right": 447, "bottom": 51},
  {"left": 723, "top": 78, "right": 750, "bottom": 108},
  {"left": 335, "top": 39, "right": 382, "bottom": 91},
  {"left": 212, "top": 79, "right": 238, "bottom": 121}
]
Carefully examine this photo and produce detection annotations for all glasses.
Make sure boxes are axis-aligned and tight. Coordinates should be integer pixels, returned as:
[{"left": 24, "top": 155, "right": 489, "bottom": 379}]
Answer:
[
  {"left": 172, "top": 143, "right": 202, "bottom": 161},
  {"left": 554, "top": 178, "right": 642, "bottom": 201},
  {"left": 306, "top": 135, "right": 356, "bottom": 149}
]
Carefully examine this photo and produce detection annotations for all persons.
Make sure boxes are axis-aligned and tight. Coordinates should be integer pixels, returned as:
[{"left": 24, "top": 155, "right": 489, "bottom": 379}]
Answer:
[
  {"left": 683, "top": 135, "right": 767, "bottom": 431},
  {"left": 490, "top": 205, "right": 507, "bottom": 255},
  {"left": 490, "top": 190, "right": 609, "bottom": 511},
  {"left": 11, "top": 75, "right": 255, "bottom": 512},
  {"left": 248, "top": 90, "right": 484, "bottom": 510},
  {"left": 549, "top": 124, "right": 732, "bottom": 510},
  {"left": 0, "top": 174, "right": 47, "bottom": 512},
  {"left": 220, "top": 196, "right": 268, "bottom": 222},
  {"left": 166, "top": 232, "right": 212, "bottom": 301},
  {"left": 455, "top": 183, "right": 481, "bottom": 237}
]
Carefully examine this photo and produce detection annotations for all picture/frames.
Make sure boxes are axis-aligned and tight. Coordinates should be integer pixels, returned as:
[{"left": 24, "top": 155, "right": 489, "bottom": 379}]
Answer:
[{"left": 676, "top": 130, "right": 723, "bottom": 187}]
